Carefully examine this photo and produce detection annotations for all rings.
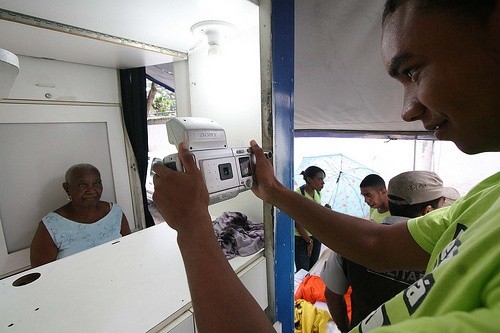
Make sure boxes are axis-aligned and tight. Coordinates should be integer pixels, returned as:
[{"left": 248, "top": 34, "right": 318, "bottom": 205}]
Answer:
[{"left": 309, "top": 252, "right": 311, "bottom": 254}]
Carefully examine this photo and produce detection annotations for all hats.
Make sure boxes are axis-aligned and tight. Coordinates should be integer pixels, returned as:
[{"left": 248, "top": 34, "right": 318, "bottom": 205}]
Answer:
[{"left": 386, "top": 171, "right": 461, "bottom": 206}]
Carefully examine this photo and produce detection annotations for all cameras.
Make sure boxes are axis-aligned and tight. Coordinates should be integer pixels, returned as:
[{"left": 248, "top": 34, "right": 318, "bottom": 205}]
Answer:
[{"left": 162, "top": 118, "right": 272, "bottom": 205}]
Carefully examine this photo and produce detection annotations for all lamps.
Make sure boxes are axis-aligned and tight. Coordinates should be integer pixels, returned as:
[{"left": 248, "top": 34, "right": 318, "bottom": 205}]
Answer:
[{"left": 191, "top": 21, "right": 236, "bottom": 60}]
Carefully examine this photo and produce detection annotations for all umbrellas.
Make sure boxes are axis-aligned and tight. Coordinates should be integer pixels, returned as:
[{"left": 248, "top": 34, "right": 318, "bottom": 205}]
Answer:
[{"left": 294, "top": 154, "right": 381, "bottom": 220}]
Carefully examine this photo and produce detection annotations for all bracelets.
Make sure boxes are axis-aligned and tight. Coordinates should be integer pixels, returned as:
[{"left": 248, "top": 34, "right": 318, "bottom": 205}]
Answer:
[{"left": 308, "top": 239, "right": 312, "bottom": 244}]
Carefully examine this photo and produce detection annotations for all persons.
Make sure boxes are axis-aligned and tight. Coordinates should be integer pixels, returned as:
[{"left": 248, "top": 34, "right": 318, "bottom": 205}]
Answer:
[
  {"left": 153, "top": 0, "right": 500, "bottom": 333},
  {"left": 293, "top": 166, "right": 332, "bottom": 272},
  {"left": 30, "top": 164, "right": 132, "bottom": 268},
  {"left": 322, "top": 171, "right": 464, "bottom": 333}
]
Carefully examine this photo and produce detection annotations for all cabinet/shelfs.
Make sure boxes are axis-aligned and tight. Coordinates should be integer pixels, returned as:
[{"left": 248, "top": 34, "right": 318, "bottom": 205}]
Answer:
[{"left": 0, "top": 220, "right": 269, "bottom": 333}]
